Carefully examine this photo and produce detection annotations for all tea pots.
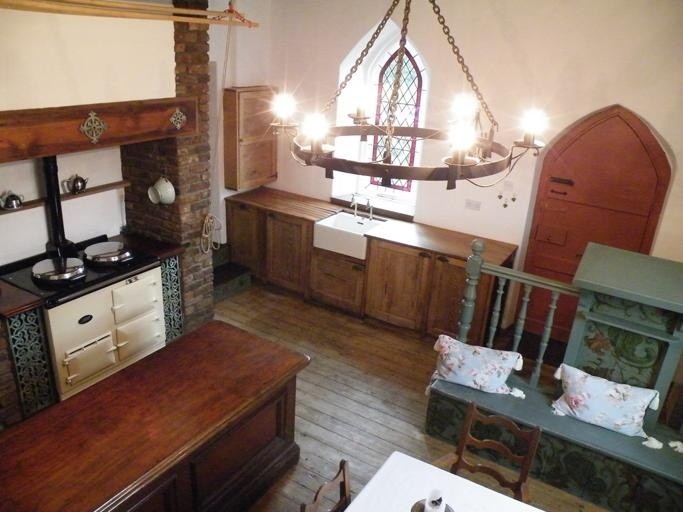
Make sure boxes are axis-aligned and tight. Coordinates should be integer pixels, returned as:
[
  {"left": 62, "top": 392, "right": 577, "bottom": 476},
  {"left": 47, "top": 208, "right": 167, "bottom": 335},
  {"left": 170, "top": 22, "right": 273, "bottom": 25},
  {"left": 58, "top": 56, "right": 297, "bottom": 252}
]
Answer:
[{"left": 61, "top": 174, "right": 89, "bottom": 194}]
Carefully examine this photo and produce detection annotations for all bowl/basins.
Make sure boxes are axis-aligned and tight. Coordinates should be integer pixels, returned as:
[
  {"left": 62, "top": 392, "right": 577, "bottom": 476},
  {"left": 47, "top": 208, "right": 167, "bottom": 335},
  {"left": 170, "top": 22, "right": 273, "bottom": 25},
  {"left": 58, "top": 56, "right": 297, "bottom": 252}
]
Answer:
[{"left": 147, "top": 177, "right": 175, "bottom": 205}]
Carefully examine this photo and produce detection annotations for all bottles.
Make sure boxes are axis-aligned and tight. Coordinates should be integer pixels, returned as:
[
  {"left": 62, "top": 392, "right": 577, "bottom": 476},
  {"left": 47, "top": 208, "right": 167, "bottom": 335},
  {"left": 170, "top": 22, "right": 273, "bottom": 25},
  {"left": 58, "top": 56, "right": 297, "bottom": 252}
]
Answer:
[{"left": 3, "top": 195, "right": 22, "bottom": 210}]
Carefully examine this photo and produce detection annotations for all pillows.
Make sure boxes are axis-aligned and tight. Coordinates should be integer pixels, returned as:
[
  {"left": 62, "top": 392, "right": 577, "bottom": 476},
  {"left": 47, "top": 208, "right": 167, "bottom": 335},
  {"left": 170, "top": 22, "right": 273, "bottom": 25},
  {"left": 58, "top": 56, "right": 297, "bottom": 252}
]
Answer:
[
  {"left": 430, "top": 334, "right": 527, "bottom": 400},
  {"left": 549, "top": 362, "right": 660, "bottom": 440}
]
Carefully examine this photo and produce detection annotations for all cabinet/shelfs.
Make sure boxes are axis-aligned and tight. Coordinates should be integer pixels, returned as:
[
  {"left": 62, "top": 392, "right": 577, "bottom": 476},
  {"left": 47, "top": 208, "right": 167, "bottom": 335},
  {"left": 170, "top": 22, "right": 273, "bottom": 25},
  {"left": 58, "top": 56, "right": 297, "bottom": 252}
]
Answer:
[
  {"left": 222, "top": 85, "right": 279, "bottom": 191},
  {"left": 226, "top": 199, "right": 313, "bottom": 296},
  {"left": 1, "top": 318, "right": 314, "bottom": 512},
  {"left": 364, "top": 233, "right": 517, "bottom": 348},
  {"left": 307, "top": 247, "right": 364, "bottom": 320}
]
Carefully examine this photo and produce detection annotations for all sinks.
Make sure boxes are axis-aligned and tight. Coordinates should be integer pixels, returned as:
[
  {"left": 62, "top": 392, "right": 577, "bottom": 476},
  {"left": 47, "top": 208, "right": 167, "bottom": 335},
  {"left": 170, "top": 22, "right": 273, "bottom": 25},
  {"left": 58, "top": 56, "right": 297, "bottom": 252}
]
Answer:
[{"left": 313, "top": 209, "right": 388, "bottom": 261}]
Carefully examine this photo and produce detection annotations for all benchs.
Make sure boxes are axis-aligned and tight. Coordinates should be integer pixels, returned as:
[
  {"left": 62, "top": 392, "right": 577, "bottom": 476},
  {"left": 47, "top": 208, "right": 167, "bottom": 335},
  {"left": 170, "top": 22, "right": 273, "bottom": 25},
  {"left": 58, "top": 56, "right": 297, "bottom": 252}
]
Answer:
[{"left": 422, "top": 372, "right": 682, "bottom": 512}]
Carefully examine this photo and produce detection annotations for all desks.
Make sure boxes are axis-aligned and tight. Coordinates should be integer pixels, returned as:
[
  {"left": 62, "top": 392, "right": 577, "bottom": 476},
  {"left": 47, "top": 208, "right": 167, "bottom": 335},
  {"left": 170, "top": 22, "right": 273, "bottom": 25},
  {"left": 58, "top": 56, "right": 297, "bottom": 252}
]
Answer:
[{"left": 343, "top": 450, "right": 547, "bottom": 512}]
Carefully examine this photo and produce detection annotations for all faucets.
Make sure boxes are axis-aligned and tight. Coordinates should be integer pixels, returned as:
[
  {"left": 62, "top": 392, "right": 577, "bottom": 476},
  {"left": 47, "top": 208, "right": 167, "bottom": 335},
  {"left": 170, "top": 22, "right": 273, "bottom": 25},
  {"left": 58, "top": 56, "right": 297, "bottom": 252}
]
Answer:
[
  {"left": 365, "top": 199, "right": 373, "bottom": 219},
  {"left": 349, "top": 194, "right": 357, "bottom": 216}
]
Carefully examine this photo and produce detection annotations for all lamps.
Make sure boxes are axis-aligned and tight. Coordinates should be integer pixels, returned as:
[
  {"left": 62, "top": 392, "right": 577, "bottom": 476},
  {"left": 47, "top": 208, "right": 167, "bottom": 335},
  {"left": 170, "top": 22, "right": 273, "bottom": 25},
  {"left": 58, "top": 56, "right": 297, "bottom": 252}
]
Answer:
[{"left": 266, "top": 0, "right": 553, "bottom": 194}]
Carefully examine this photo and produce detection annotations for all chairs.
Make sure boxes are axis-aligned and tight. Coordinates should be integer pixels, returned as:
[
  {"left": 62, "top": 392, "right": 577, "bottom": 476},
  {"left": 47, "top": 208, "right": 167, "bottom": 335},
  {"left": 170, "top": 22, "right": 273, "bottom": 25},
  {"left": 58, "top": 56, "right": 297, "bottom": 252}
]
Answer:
[
  {"left": 298, "top": 459, "right": 352, "bottom": 512},
  {"left": 446, "top": 403, "right": 542, "bottom": 504}
]
